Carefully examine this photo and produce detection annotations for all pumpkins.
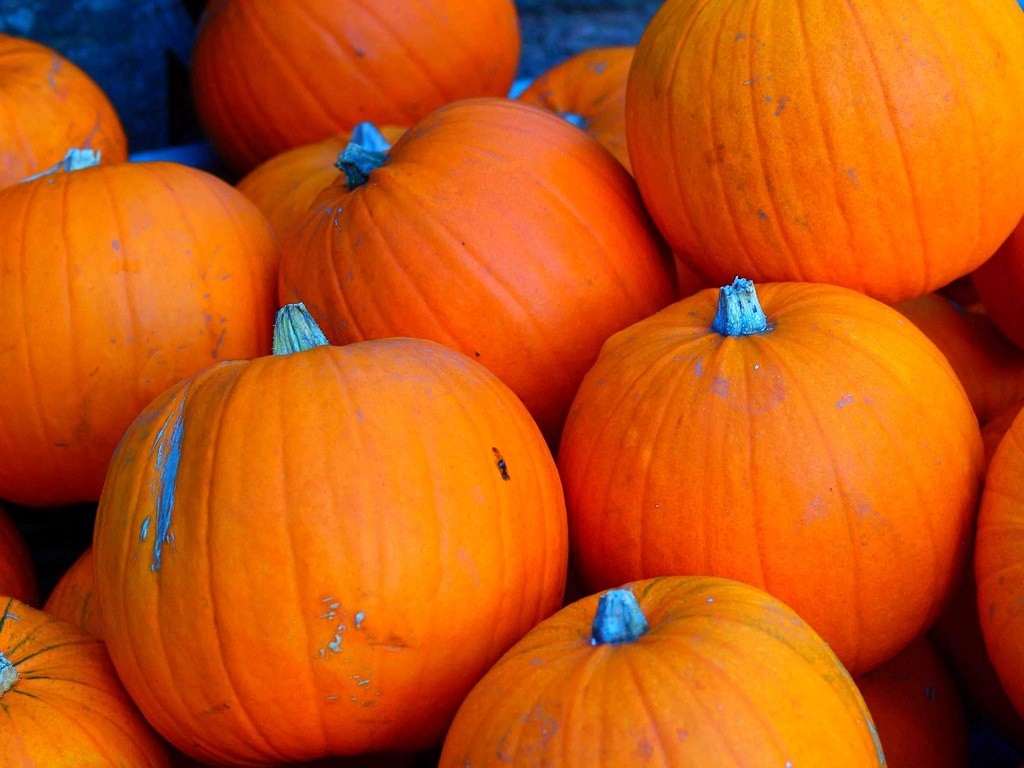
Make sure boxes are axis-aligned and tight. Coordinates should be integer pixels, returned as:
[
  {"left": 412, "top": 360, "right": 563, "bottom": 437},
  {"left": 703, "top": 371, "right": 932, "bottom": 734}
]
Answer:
[{"left": 0, "top": 0, "right": 1024, "bottom": 767}]
